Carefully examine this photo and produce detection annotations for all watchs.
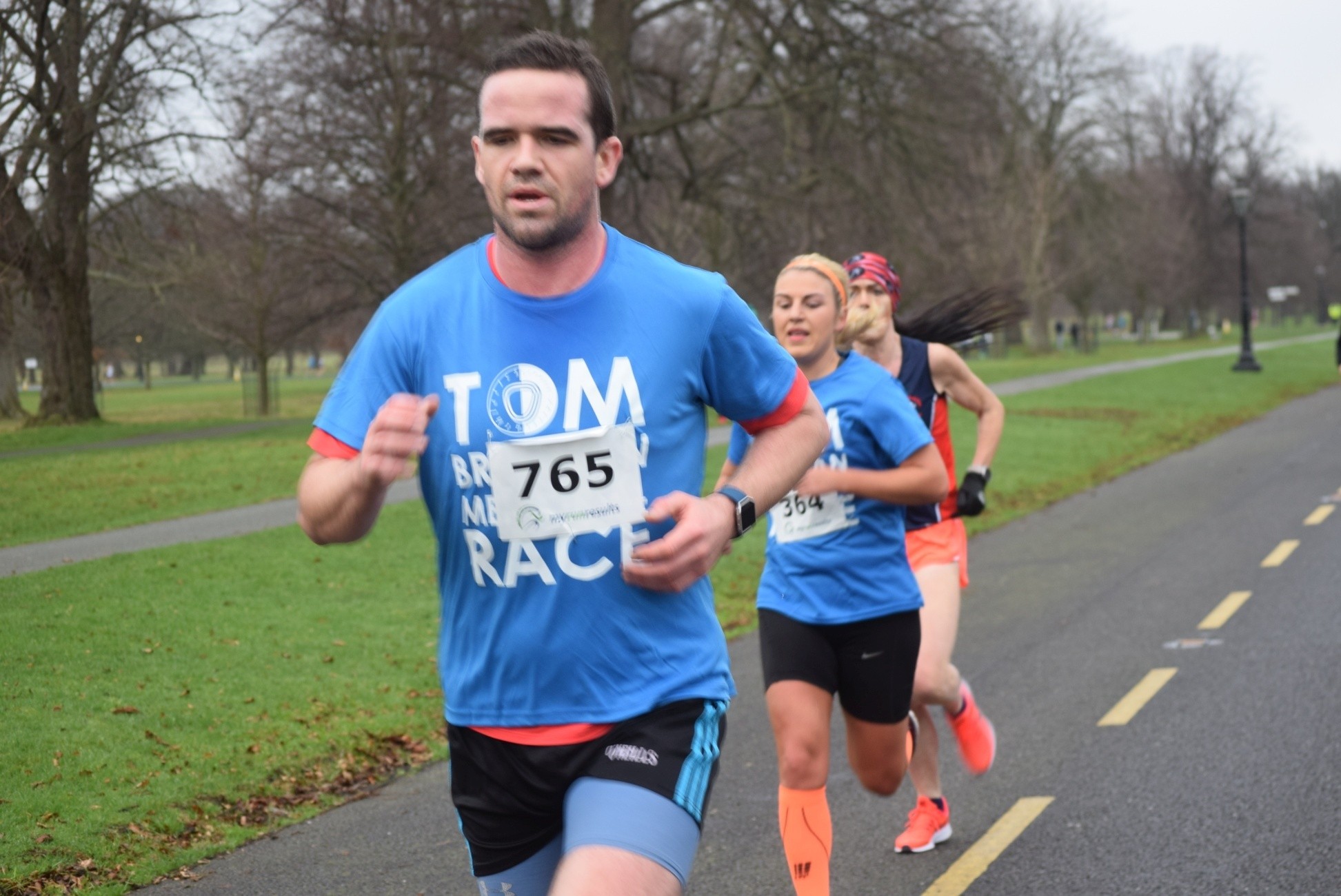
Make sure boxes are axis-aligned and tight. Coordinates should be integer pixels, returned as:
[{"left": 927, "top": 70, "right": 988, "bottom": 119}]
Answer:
[
  {"left": 708, "top": 484, "right": 759, "bottom": 539},
  {"left": 967, "top": 462, "right": 991, "bottom": 481}
]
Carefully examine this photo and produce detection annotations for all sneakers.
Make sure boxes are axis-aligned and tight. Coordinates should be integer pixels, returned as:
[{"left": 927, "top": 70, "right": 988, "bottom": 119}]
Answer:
[
  {"left": 892, "top": 795, "right": 952, "bottom": 854},
  {"left": 949, "top": 678, "right": 993, "bottom": 778}
]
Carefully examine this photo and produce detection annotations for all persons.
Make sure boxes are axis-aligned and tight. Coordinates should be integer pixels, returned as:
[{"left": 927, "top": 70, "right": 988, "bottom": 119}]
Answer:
[
  {"left": 713, "top": 256, "right": 950, "bottom": 896},
  {"left": 1055, "top": 318, "right": 1079, "bottom": 348},
  {"left": 299, "top": 34, "right": 828, "bottom": 896},
  {"left": 843, "top": 252, "right": 1005, "bottom": 856}
]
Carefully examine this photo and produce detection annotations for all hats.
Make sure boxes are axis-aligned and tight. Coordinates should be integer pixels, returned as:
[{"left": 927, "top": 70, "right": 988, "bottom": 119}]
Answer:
[{"left": 844, "top": 252, "right": 902, "bottom": 310}]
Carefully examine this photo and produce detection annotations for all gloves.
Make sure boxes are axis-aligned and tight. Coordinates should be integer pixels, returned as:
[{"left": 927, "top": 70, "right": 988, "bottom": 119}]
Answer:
[{"left": 953, "top": 471, "right": 985, "bottom": 517}]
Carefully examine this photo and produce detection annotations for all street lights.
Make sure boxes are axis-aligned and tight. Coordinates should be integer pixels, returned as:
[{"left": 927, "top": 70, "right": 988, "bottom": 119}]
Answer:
[{"left": 1228, "top": 186, "right": 1262, "bottom": 371}]
